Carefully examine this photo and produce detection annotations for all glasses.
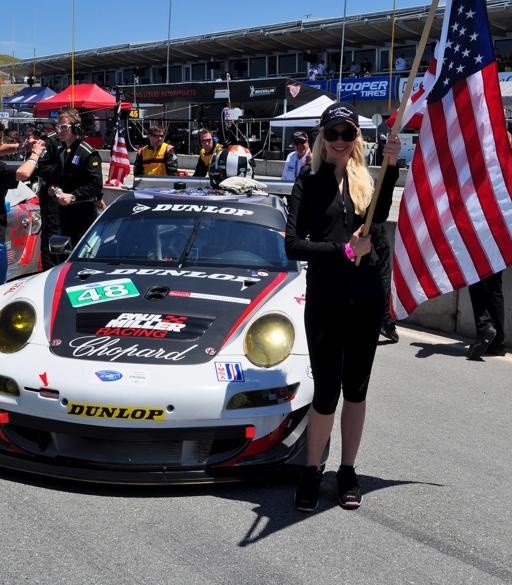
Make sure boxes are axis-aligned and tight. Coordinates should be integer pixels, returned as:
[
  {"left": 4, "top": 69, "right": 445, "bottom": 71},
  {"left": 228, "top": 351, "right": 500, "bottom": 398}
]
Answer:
[
  {"left": 56, "top": 124, "right": 73, "bottom": 130},
  {"left": 323, "top": 128, "right": 355, "bottom": 142}
]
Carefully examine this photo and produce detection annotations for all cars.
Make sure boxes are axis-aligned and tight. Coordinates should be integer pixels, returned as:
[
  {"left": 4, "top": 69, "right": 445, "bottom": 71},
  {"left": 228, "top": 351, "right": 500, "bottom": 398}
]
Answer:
[
  {"left": 18, "top": 111, "right": 32, "bottom": 117},
  {"left": 36, "top": 127, "right": 104, "bottom": 151}
]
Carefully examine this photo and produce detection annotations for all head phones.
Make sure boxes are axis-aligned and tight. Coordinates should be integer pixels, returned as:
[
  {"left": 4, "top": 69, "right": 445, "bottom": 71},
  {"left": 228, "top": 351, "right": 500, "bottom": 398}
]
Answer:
[{"left": 65, "top": 111, "right": 84, "bottom": 136}]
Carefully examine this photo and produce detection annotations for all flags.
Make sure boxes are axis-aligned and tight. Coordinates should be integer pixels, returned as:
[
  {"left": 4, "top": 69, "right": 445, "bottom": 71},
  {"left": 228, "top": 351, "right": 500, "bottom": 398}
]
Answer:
[
  {"left": 385, "top": 0, "right": 512, "bottom": 321},
  {"left": 105, "top": 103, "right": 130, "bottom": 187}
]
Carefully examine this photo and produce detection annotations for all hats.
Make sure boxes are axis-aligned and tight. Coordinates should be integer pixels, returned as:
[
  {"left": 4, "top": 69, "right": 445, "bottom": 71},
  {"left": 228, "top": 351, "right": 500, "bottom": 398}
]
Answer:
[
  {"left": 320, "top": 104, "right": 360, "bottom": 134},
  {"left": 292, "top": 132, "right": 308, "bottom": 144}
]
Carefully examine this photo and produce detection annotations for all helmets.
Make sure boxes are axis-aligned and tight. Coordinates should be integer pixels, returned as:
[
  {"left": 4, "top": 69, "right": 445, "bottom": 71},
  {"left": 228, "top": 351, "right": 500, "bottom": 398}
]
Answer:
[{"left": 209, "top": 144, "right": 256, "bottom": 190}]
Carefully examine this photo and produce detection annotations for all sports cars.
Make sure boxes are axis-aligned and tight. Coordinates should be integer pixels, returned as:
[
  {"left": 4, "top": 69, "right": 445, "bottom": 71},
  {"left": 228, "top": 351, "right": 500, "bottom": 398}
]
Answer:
[
  {"left": 3, "top": 180, "right": 43, "bottom": 285},
  {"left": 0, "top": 175, "right": 315, "bottom": 490}
]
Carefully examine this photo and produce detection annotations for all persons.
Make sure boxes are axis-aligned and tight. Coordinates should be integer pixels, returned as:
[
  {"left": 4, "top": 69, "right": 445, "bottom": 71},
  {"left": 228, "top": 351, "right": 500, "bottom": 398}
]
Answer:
[
  {"left": 192, "top": 129, "right": 225, "bottom": 177},
  {"left": 0, "top": 109, "right": 108, "bottom": 284},
  {"left": 283, "top": 101, "right": 402, "bottom": 512},
  {"left": 372, "top": 224, "right": 399, "bottom": 342},
  {"left": 466, "top": 271, "right": 505, "bottom": 361},
  {"left": 303, "top": 52, "right": 414, "bottom": 86},
  {"left": 280, "top": 131, "right": 312, "bottom": 181},
  {"left": 133, "top": 125, "right": 179, "bottom": 176}
]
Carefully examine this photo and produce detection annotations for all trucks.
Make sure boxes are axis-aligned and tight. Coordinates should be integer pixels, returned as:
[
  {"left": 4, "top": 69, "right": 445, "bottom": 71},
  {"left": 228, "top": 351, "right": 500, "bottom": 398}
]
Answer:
[
  {"left": 0, "top": 112, "right": 9, "bottom": 120},
  {"left": 388, "top": 132, "right": 420, "bottom": 169},
  {"left": 3, "top": 109, "right": 18, "bottom": 117},
  {"left": 7, "top": 118, "right": 58, "bottom": 143}
]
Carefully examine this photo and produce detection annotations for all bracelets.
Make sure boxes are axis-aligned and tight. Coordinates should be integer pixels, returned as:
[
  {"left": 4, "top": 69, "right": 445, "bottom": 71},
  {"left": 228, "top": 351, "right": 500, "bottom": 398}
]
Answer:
[{"left": 344, "top": 241, "right": 356, "bottom": 260}]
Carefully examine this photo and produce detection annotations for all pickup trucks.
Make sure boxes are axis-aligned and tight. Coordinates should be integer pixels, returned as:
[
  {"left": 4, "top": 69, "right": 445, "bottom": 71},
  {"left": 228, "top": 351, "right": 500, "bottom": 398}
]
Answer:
[{"left": 362, "top": 136, "right": 376, "bottom": 158}]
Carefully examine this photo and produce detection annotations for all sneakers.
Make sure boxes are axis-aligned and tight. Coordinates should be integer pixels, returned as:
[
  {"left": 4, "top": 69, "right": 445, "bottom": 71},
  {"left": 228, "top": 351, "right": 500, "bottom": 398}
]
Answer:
[
  {"left": 293, "top": 464, "right": 323, "bottom": 512},
  {"left": 380, "top": 325, "right": 398, "bottom": 342},
  {"left": 336, "top": 465, "right": 362, "bottom": 507}
]
